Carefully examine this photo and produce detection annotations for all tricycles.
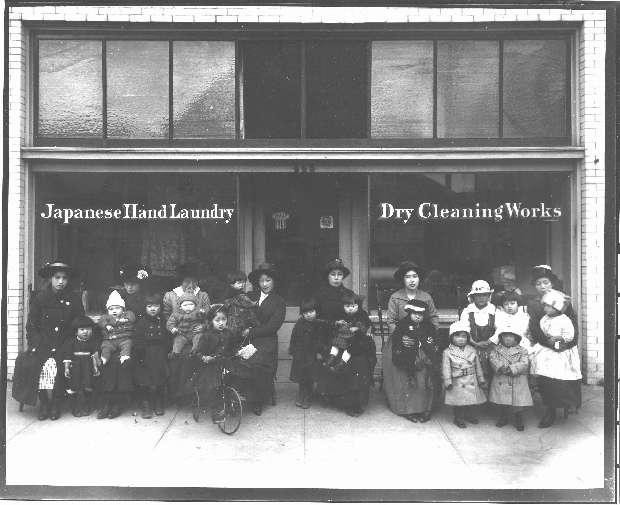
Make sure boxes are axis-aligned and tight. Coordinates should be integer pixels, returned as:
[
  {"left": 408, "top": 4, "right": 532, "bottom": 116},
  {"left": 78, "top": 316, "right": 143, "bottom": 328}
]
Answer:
[{"left": 191, "top": 347, "right": 245, "bottom": 438}]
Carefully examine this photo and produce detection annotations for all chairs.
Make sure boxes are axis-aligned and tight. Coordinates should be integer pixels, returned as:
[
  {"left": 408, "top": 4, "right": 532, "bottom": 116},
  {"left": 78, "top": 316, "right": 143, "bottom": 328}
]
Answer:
[
  {"left": 20, "top": 280, "right": 84, "bottom": 413},
  {"left": 377, "top": 302, "right": 442, "bottom": 394}
]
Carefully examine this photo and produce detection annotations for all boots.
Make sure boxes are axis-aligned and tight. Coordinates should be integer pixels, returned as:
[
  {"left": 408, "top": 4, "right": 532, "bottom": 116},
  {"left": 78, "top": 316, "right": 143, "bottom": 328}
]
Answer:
[
  {"left": 300, "top": 383, "right": 315, "bottom": 410},
  {"left": 294, "top": 381, "right": 306, "bottom": 408}
]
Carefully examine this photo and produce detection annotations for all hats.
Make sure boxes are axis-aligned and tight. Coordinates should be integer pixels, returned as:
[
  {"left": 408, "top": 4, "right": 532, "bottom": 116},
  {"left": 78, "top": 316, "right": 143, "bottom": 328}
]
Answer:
[
  {"left": 246, "top": 260, "right": 286, "bottom": 290},
  {"left": 321, "top": 253, "right": 352, "bottom": 281},
  {"left": 70, "top": 314, "right": 95, "bottom": 330},
  {"left": 37, "top": 255, "right": 74, "bottom": 280},
  {"left": 391, "top": 259, "right": 431, "bottom": 286},
  {"left": 174, "top": 261, "right": 205, "bottom": 284},
  {"left": 448, "top": 319, "right": 472, "bottom": 337},
  {"left": 119, "top": 261, "right": 154, "bottom": 283},
  {"left": 464, "top": 278, "right": 496, "bottom": 299},
  {"left": 142, "top": 292, "right": 164, "bottom": 306},
  {"left": 539, "top": 287, "right": 573, "bottom": 314},
  {"left": 402, "top": 298, "right": 430, "bottom": 313},
  {"left": 102, "top": 289, "right": 127, "bottom": 310},
  {"left": 176, "top": 288, "right": 200, "bottom": 307},
  {"left": 494, "top": 319, "right": 524, "bottom": 341}
]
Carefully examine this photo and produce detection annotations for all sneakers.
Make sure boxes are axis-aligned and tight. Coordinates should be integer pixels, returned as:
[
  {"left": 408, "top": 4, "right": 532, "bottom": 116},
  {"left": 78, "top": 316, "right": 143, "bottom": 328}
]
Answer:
[
  {"left": 495, "top": 413, "right": 511, "bottom": 429},
  {"left": 466, "top": 414, "right": 480, "bottom": 426},
  {"left": 70, "top": 406, "right": 81, "bottom": 418},
  {"left": 96, "top": 404, "right": 112, "bottom": 420},
  {"left": 37, "top": 401, "right": 49, "bottom": 422},
  {"left": 536, "top": 408, "right": 560, "bottom": 429},
  {"left": 48, "top": 400, "right": 64, "bottom": 421},
  {"left": 106, "top": 405, "right": 123, "bottom": 419},
  {"left": 78, "top": 407, "right": 90, "bottom": 418},
  {"left": 252, "top": 402, "right": 264, "bottom": 417},
  {"left": 452, "top": 416, "right": 468, "bottom": 430},
  {"left": 141, "top": 400, "right": 154, "bottom": 420},
  {"left": 320, "top": 352, "right": 339, "bottom": 369},
  {"left": 418, "top": 411, "right": 432, "bottom": 423},
  {"left": 507, "top": 413, "right": 528, "bottom": 433},
  {"left": 406, "top": 413, "right": 419, "bottom": 424},
  {"left": 152, "top": 398, "right": 167, "bottom": 416},
  {"left": 327, "top": 356, "right": 350, "bottom": 378}
]
